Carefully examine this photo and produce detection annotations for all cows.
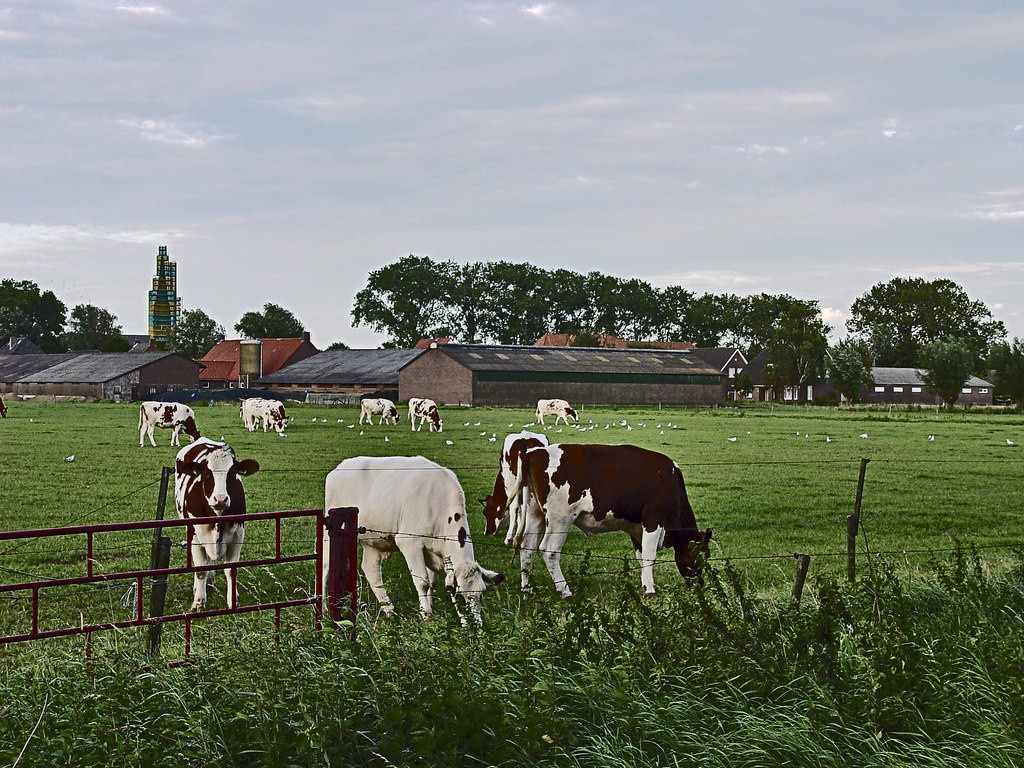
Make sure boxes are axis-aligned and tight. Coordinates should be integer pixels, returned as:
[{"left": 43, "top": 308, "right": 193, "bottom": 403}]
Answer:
[
  {"left": 174, "top": 436, "right": 260, "bottom": 613},
  {"left": 239, "top": 399, "right": 285, "bottom": 433},
  {"left": 358, "top": 398, "right": 401, "bottom": 426},
  {"left": 322, "top": 454, "right": 505, "bottom": 632},
  {"left": 506, "top": 443, "right": 713, "bottom": 599},
  {"left": 535, "top": 399, "right": 580, "bottom": 426},
  {"left": 477, "top": 430, "right": 549, "bottom": 536},
  {"left": 407, "top": 398, "right": 443, "bottom": 433},
  {"left": 138, "top": 401, "right": 202, "bottom": 447},
  {"left": 0, "top": 397, "right": 9, "bottom": 418},
  {"left": 239, "top": 397, "right": 289, "bottom": 428}
]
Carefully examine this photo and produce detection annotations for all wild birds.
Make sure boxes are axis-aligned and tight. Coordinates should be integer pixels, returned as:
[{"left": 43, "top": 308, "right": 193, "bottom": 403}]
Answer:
[
  {"left": 63, "top": 455, "right": 76, "bottom": 462},
  {"left": 1006, "top": 439, "right": 1014, "bottom": 445},
  {"left": 220, "top": 415, "right": 936, "bottom": 445}
]
